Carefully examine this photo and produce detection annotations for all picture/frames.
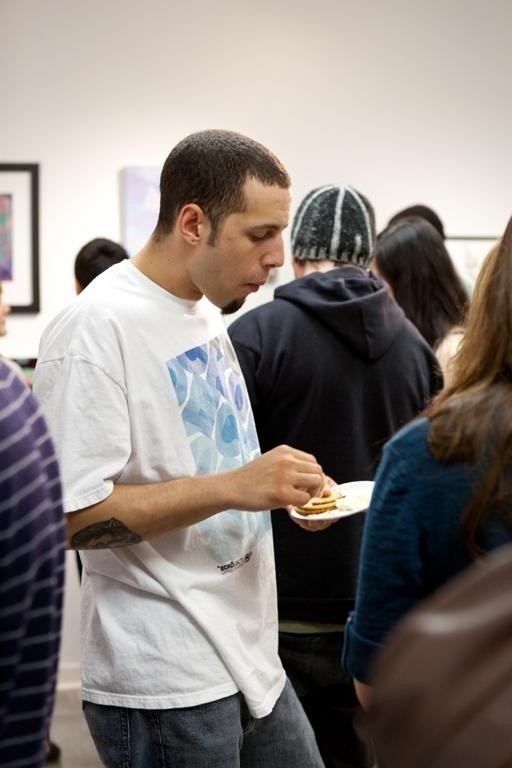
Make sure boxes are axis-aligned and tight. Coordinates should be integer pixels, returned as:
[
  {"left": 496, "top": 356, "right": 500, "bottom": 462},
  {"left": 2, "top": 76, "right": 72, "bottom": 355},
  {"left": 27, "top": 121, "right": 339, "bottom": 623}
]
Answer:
[{"left": 0, "top": 163, "right": 42, "bottom": 314}]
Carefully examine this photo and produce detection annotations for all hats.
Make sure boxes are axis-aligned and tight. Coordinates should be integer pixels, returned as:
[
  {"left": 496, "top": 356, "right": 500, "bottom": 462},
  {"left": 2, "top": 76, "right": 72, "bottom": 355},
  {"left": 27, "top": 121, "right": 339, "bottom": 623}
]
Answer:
[{"left": 287, "top": 182, "right": 380, "bottom": 271}]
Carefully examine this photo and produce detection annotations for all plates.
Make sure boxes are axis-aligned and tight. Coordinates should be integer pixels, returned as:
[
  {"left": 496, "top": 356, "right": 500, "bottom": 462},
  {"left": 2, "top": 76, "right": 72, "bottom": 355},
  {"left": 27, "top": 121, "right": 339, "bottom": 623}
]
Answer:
[{"left": 290, "top": 479, "right": 376, "bottom": 521}]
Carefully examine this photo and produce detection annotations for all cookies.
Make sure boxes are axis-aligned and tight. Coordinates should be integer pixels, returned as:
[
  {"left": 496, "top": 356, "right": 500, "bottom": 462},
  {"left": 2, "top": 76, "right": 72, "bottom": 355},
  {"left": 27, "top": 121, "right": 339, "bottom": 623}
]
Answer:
[{"left": 294, "top": 494, "right": 346, "bottom": 515}]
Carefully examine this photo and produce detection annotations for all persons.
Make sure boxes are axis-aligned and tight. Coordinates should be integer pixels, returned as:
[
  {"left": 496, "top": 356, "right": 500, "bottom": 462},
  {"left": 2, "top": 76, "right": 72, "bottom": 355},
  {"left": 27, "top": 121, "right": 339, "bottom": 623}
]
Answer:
[
  {"left": 73, "top": 237, "right": 131, "bottom": 296},
  {"left": 1, "top": 282, "right": 13, "bottom": 338},
  {"left": 28, "top": 126, "right": 338, "bottom": 768},
  {"left": 342, "top": 215, "right": 511, "bottom": 713},
  {"left": 371, "top": 217, "right": 473, "bottom": 396},
  {"left": 220, "top": 184, "right": 446, "bottom": 767},
  {"left": 0, "top": 352, "right": 66, "bottom": 767},
  {"left": 388, "top": 201, "right": 450, "bottom": 238}
]
